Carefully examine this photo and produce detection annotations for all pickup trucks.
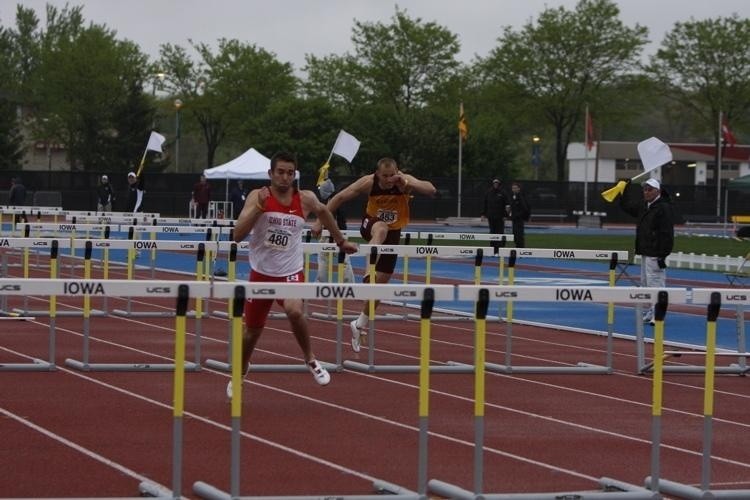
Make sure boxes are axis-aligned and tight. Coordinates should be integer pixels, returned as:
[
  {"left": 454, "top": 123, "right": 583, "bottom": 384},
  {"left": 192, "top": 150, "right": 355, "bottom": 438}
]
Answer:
[{"left": 729, "top": 215, "right": 750, "bottom": 238}]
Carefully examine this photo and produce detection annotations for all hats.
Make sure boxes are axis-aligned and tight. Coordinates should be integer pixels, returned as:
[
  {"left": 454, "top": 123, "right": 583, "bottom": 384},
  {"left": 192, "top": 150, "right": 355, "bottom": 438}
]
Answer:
[{"left": 641, "top": 178, "right": 660, "bottom": 190}]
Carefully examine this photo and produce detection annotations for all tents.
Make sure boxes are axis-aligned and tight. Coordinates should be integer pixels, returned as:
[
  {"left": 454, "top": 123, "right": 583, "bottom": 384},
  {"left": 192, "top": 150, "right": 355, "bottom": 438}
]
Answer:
[{"left": 203, "top": 147, "right": 300, "bottom": 219}]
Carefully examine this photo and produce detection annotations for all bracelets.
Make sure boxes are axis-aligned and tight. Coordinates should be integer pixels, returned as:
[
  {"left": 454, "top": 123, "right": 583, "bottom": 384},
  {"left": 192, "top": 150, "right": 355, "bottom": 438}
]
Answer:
[
  {"left": 256, "top": 202, "right": 265, "bottom": 212},
  {"left": 336, "top": 239, "right": 345, "bottom": 246},
  {"left": 401, "top": 179, "right": 407, "bottom": 187}
]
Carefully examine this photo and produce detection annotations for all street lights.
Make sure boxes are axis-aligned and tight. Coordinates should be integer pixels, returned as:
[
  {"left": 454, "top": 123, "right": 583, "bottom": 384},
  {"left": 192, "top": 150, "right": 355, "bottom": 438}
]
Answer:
[
  {"left": 151, "top": 72, "right": 165, "bottom": 130},
  {"left": 531, "top": 134, "right": 540, "bottom": 180},
  {"left": 173, "top": 99, "right": 183, "bottom": 174}
]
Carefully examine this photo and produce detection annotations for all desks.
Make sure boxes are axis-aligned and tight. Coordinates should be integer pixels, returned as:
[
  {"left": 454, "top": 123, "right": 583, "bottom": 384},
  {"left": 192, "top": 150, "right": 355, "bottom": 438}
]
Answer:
[{"left": 209, "top": 201, "right": 233, "bottom": 219}]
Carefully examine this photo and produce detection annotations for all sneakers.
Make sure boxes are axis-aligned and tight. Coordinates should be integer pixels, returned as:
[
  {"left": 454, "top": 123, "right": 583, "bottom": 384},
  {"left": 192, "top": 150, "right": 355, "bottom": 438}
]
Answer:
[
  {"left": 350, "top": 320, "right": 362, "bottom": 353},
  {"left": 227, "top": 362, "right": 250, "bottom": 400},
  {"left": 305, "top": 355, "right": 330, "bottom": 386}
]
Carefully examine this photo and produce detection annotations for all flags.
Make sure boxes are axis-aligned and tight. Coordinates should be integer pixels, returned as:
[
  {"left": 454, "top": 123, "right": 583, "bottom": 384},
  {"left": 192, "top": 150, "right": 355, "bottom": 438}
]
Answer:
[
  {"left": 333, "top": 129, "right": 362, "bottom": 163},
  {"left": 457, "top": 100, "right": 469, "bottom": 146},
  {"left": 585, "top": 105, "right": 595, "bottom": 151},
  {"left": 720, "top": 113, "right": 737, "bottom": 145},
  {"left": 147, "top": 130, "right": 167, "bottom": 153}
]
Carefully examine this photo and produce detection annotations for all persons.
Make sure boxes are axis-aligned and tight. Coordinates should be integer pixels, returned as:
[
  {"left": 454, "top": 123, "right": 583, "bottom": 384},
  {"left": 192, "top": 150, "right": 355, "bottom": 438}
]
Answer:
[
  {"left": 95, "top": 175, "right": 117, "bottom": 212},
  {"left": 218, "top": 205, "right": 224, "bottom": 219},
  {"left": 618, "top": 177, "right": 673, "bottom": 326},
  {"left": 480, "top": 179, "right": 511, "bottom": 254},
  {"left": 193, "top": 174, "right": 213, "bottom": 218},
  {"left": 125, "top": 171, "right": 147, "bottom": 211},
  {"left": 8, "top": 177, "right": 29, "bottom": 223},
  {"left": 234, "top": 180, "right": 252, "bottom": 219},
  {"left": 317, "top": 164, "right": 346, "bottom": 284},
  {"left": 507, "top": 182, "right": 529, "bottom": 248},
  {"left": 225, "top": 152, "right": 359, "bottom": 402},
  {"left": 310, "top": 158, "right": 436, "bottom": 352}
]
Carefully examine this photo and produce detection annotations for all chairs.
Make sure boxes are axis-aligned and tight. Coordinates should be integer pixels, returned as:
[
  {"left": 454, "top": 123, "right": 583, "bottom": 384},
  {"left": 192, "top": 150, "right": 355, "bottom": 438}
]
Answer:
[
  {"left": 188, "top": 191, "right": 202, "bottom": 219},
  {"left": 208, "top": 201, "right": 218, "bottom": 219},
  {"left": 723, "top": 253, "right": 750, "bottom": 289},
  {"left": 614, "top": 249, "right": 641, "bottom": 288}
]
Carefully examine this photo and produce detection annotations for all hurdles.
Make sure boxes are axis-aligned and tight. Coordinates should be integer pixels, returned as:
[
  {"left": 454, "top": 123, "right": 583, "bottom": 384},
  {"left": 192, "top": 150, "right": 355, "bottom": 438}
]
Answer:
[{"left": 0, "top": 205, "right": 750, "bottom": 500}]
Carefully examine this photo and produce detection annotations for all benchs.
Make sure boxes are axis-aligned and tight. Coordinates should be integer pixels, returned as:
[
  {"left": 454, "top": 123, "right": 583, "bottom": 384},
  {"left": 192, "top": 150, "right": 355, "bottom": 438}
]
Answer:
[{"left": 528, "top": 208, "right": 569, "bottom": 222}]
